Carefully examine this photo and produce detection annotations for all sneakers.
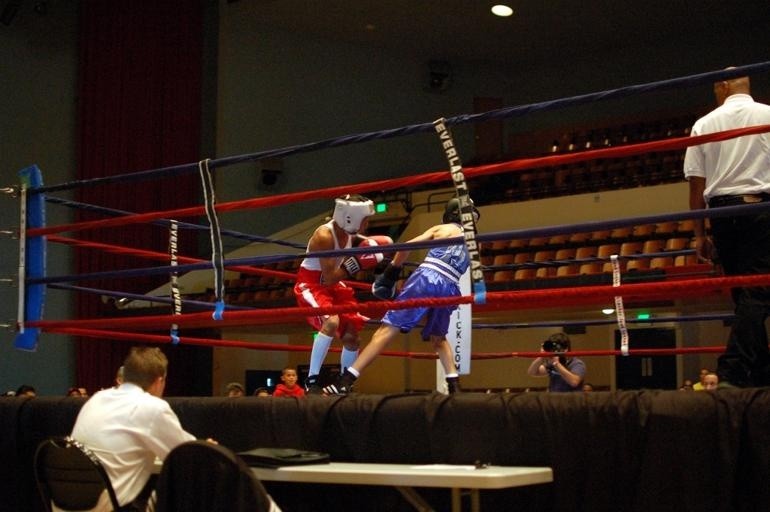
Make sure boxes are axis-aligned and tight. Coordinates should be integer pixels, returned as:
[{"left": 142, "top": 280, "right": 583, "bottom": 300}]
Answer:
[
  {"left": 304, "top": 376, "right": 353, "bottom": 396},
  {"left": 448, "top": 382, "right": 462, "bottom": 393}
]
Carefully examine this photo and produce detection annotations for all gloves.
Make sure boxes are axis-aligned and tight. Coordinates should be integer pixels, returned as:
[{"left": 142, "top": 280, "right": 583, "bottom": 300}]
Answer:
[
  {"left": 372, "top": 262, "right": 402, "bottom": 300},
  {"left": 340, "top": 235, "right": 394, "bottom": 275}
]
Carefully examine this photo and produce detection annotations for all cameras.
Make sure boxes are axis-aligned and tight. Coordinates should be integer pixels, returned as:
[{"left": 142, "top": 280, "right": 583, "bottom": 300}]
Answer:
[{"left": 543, "top": 341, "right": 567, "bottom": 364}]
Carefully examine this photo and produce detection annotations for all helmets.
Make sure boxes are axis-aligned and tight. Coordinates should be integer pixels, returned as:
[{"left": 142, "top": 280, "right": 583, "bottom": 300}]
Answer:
[
  {"left": 443, "top": 197, "right": 480, "bottom": 224},
  {"left": 332, "top": 198, "right": 376, "bottom": 235}
]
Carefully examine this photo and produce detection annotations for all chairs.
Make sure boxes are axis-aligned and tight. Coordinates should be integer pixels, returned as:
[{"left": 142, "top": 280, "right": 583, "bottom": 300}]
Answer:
[
  {"left": 478, "top": 217, "right": 715, "bottom": 280},
  {"left": 197, "top": 261, "right": 296, "bottom": 302},
  {"left": 34, "top": 436, "right": 269, "bottom": 512}
]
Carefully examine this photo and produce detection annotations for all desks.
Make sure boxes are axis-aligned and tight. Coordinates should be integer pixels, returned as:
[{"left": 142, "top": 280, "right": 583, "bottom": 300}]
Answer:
[{"left": 152, "top": 458, "right": 553, "bottom": 511}]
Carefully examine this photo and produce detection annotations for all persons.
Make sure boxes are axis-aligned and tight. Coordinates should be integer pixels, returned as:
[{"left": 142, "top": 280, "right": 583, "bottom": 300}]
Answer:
[
  {"left": 684, "top": 66, "right": 770, "bottom": 389},
  {"left": 293, "top": 193, "right": 395, "bottom": 396},
  {"left": 226, "top": 383, "right": 245, "bottom": 397},
  {"left": 15, "top": 385, "right": 36, "bottom": 396},
  {"left": 681, "top": 368, "right": 718, "bottom": 391},
  {"left": 68, "top": 386, "right": 81, "bottom": 397},
  {"left": 115, "top": 366, "right": 124, "bottom": 385},
  {"left": 78, "top": 387, "right": 89, "bottom": 397},
  {"left": 320, "top": 197, "right": 482, "bottom": 396},
  {"left": 584, "top": 383, "right": 594, "bottom": 392},
  {"left": 527, "top": 334, "right": 586, "bottom": 393},
  {"left": 273, "top": 364, "right": 305, "bottom": 398},
  {"left": 50, "top": 345, "right": 219, "bottom": 512},
  {"left": 254, "top": 387, "right": 273, "bottom": 396}
]
